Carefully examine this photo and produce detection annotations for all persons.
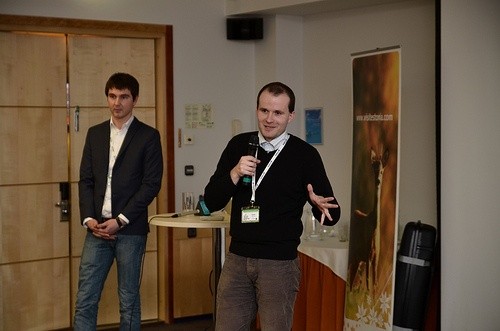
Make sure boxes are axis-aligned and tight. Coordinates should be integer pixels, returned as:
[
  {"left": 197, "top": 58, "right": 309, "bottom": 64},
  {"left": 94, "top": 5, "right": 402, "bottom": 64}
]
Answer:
[
  {"left": 74, "top": 72, "right": 163, "bottom": 331},
  {"left": 204, "top": 82, "right": 341, "bottom": 331}
]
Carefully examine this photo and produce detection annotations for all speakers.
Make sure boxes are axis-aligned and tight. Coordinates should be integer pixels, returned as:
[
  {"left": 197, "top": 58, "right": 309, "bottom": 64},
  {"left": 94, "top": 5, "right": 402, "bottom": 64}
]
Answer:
[{"left": 226, "top": 16, "right": 264, "bottom": 41}]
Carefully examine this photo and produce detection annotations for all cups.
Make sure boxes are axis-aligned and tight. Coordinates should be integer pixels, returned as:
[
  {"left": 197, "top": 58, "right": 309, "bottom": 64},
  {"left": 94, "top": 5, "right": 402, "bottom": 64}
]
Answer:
[
  {"left": 305, "top": 209, "right": 320, "bottom": 238},
  {"left": 338, "top": 223, "right": 347, "bottom": 242},
  {"left": 182, "top": 193, "right": 193, "bottom": 214}
]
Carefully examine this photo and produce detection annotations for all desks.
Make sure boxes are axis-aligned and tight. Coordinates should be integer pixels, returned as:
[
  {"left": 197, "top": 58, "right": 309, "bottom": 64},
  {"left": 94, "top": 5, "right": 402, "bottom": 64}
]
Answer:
[
  {"left": 148, "top": 211, "right": 230, "bottom": 331},
  {"left": 256, "top": 232, "right": 350, "bottom": 330}
]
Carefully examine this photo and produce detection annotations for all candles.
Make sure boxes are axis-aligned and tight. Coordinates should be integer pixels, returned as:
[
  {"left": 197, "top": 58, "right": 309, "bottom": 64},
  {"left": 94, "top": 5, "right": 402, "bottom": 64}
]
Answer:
[{"left": 312, "top": 215, "right": 316, "bottom": 234}]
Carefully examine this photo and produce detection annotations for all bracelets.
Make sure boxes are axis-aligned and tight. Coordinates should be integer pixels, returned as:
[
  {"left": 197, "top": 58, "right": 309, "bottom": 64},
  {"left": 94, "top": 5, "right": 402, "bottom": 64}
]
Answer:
[{"left": 116, "top": 217, "right": 123, "bottom": 229}]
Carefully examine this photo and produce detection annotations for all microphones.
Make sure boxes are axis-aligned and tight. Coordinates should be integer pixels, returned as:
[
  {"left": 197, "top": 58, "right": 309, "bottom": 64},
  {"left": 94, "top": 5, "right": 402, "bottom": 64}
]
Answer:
[
  {"left": 172, "top": 202, "right": 211, "bottom": 218},
  {"left": 242, "top": 135, "right": 260, "bottom": 187}
]
